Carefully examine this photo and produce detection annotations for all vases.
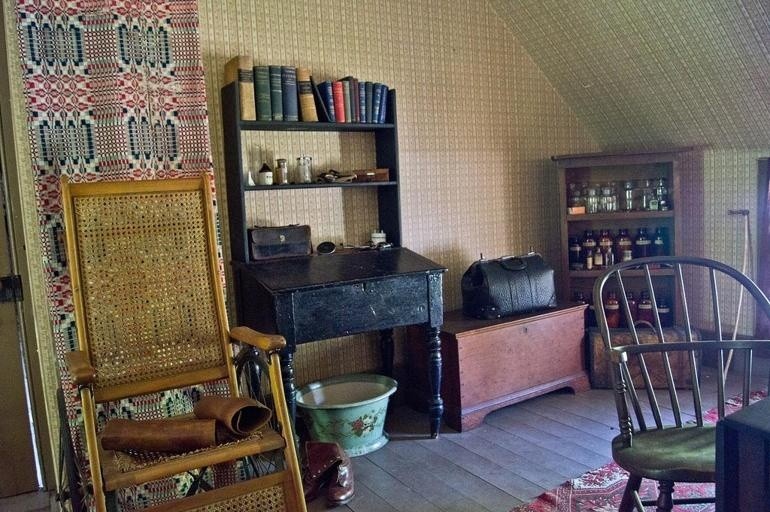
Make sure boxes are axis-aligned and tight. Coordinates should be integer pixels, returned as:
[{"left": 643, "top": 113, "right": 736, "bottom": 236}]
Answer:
[{"left": 292, "top": 372, "right": 399, "bottom": 458}]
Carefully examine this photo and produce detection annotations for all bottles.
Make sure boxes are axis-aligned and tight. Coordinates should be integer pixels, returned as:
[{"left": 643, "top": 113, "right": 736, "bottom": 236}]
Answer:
[
  {"left": 573, "top": 287, "right": 671, "bottom": 328},
  {"left": 294, "top": 156, "right": 313, "bottom": 184},
  {"left": 569, "top": 175, "right": 671, "bottom": 214},
  {"left": 275, "top": 158, "right": 289, "bottom": 185},
  {"left": 569, "top": 226, "right": 667, "bottom": 270}
]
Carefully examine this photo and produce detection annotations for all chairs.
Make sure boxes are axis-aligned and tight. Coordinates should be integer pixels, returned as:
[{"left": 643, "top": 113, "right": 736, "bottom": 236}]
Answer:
[
  {"left": 591, "top": 257, "right": 769, "bottom": 507},
  {"left": 58, "top": 182, "right": 307, "bottom": 507}
]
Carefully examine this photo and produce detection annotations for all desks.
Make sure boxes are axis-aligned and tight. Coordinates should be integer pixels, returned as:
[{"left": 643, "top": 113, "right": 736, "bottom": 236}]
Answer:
[
  {"left": 233, "top": 249, "right": 449, "bottom": 440},
  {"left": 715, "top": 398, "right": 768, "bottom": 507}
]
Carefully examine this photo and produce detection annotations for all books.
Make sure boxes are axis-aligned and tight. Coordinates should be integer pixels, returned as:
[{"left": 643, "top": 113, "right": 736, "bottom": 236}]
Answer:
[{"left": 220, "top": 53, "right": 390, "bottom": 128}]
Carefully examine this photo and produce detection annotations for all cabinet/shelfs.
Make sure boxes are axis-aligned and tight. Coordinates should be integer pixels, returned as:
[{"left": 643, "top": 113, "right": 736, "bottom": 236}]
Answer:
[
  {"left": 551, "top": 154, "right": 709, "bottom": 330},
  {"left": 221, "top": 84, "right": 400, "bottom": 264}
]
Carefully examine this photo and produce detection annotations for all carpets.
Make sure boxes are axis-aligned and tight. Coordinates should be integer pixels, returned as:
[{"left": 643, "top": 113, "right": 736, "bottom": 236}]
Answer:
[{"left": 516, "top": 391, "right": 770, "bottom": 507}]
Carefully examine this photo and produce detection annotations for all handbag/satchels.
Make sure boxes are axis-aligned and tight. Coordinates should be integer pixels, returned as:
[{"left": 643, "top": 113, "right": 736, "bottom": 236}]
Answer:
[
  {"left": 585, "top": 320, "right": 702, "bottom": 390},
  {"left": 460, "top": 251, "right": 558, "bottom": 320}
]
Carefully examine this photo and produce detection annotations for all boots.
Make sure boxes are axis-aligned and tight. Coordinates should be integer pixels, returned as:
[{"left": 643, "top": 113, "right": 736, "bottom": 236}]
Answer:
[{"left": 301, "top": 441, "right": 355, "bottom": 507}]
